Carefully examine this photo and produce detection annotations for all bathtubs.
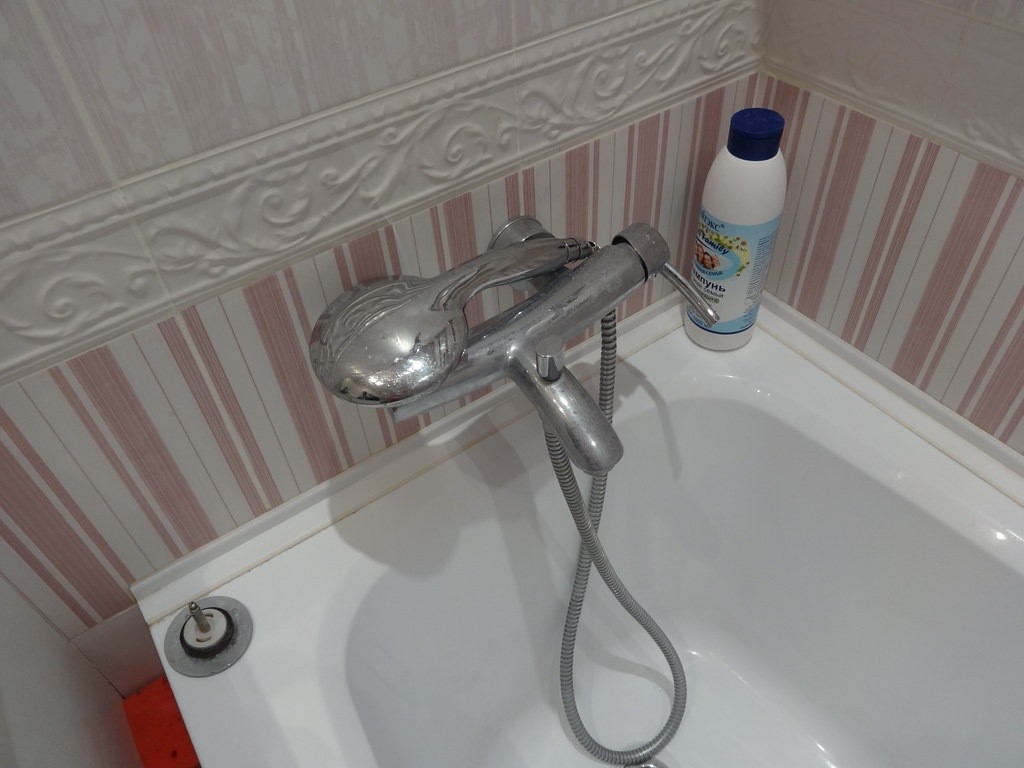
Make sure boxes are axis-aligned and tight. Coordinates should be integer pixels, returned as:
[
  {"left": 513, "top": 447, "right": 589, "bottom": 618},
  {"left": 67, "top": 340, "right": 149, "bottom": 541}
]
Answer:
[{"left": 127, "top": 281, "right": 1024, "bottom": 768}]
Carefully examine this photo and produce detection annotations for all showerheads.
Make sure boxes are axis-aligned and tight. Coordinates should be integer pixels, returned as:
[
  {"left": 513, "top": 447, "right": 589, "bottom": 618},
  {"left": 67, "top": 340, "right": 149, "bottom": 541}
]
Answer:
[{"left": 306, "top": 236, "right": 597, "bottom": 409}]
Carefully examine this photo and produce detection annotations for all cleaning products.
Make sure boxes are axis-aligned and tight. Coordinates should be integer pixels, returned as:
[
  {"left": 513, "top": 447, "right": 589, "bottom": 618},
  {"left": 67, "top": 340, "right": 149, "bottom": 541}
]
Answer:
[{"left": 682, "top": 108, "right": 789, "bottom": 353}]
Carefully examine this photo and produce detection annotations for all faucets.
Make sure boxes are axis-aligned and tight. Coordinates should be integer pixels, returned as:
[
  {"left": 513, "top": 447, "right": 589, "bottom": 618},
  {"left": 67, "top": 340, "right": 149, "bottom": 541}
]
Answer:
[{"left": 375, "top": 243, "right": 647, "bottom": 478}]
